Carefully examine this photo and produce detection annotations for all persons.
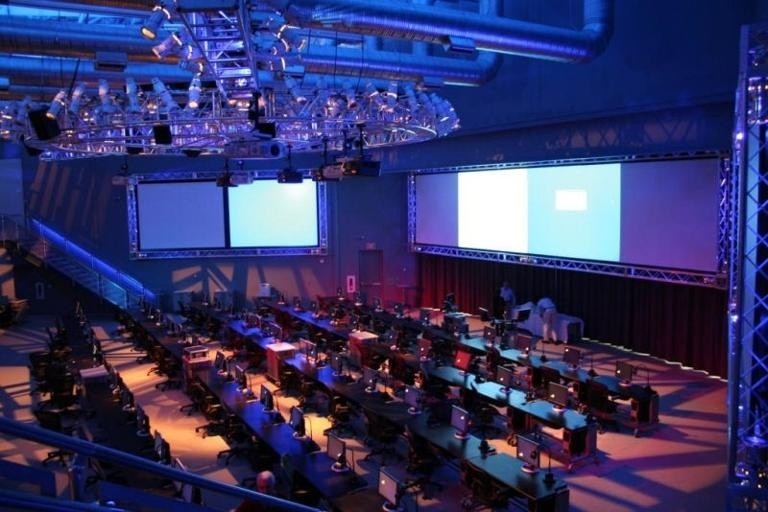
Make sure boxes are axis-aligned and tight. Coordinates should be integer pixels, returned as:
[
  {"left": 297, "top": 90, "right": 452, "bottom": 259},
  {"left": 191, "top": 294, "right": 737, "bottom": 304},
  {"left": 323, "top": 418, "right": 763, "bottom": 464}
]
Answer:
[
  {"left": 492, "top": 289, "right": 506, "bottom": 335},
  {"left": 274, "top": 453, "right": 295, "bottom": 500},
  {"left": 228, "top": 468, "right": 290, "bottom": 512},
  {"left": 537, "top": 297, "right": 561, "bottom": 345},
  {"left": 501, "top": 281, "right": 516, "bottom": 318},
  {"left": 484, "top": 342, "right": 501, "bottom": 380}
]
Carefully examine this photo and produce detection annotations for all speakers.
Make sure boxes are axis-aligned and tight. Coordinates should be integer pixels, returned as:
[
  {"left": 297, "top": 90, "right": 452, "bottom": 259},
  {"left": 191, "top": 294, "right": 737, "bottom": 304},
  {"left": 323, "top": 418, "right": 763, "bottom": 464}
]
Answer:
[
  {"left": 19, "top": 134, "right": 43, "bottom": 158},
  {"left": 181, "top": 150, "right": 202, "bottom": 158},
  {"left": 125, "top": 129, "right": 144, "bottom": 155},
  {"left": 257, "top": 122, "right": 276, "bottom": 138},
  {"left": 29, "top": 108, "right": 61, "bottom": 141},
  {"left": 151, "top": 125, "right": 172, "bottom": 145}
]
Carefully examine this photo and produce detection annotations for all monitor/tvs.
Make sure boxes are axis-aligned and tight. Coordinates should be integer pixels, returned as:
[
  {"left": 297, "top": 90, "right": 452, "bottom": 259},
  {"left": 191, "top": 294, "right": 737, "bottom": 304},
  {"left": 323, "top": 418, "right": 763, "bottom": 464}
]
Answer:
[
  {"left": 547, "top": 381, "right": 569, "bottom": 412},
  {"left": 153, "top": 430, "right": 166, "bottom": 461},
  {"left": 75, "top": 301, "right": 134, "bottom": 408},
  {"left": 298, "top": 338, "right": 318, "bottom": 361},
  {"left": 377, "top": 470, "right": 406, "bottom": 512},
  {"left": 308, "top": 300, "right": 320, "bottom": 319},
  {"left": 394, "top": 303, "right": 405, "bottom": 319},
  {"left": 373, "top": 296, "right": 384, "bottom": 312},
  {"left": 452, "top": 349, "right": 473, "bottom": 376},
  {"left": 496, "top": 365, "right": 512, "bottom": 393},
  {"left": 516, "top": 434, "right": 540, "bottom": 473},
  {"left": 419, "top": 307, "right": 433, "bottom": 326},
  {"left": 514, "top": 336, "right": 532, "bottom": 359},
  {"left": 288, "top": 406, "right": 308, "bottom": 442},
  {"left": 268, "top": 322, "right": 283, "bottom": 343},
  {"left": 214, "top": 351, "right": 229, "bottom": 377},
  {"left": 417, "top": 338, "right": 433, "bottom": 361},
  {"left": 615, "top": 361, "right": 632, "bottom": 388},
  {"left": 563, "top": 347, "right": 581, "bottom": 371},
  {"left": 214, "top": 296, "right": 262, "bottom": 329},
  {"left": 260, "top": 383, "right": 278, "bottom": 413},
  {"left": 450, "top": 404, "right": 471, "bottom": 440},
  {"left": 330, "top": 353, "right": 345, "bottom": 377},
  {"left": 404, "top": 384, "right": 422, "bottom": 415},
  {"left": 171, "top": 458, "right": 195, "bottom": 503},
  {"left": 135, "top": 403, "right": 150, "bottom": 436},
  {"left": 362, "top": 366, "right": 378, "bottom": 394},
  {"left": 292, "top": 296, "right": 303, "bottom": 312},
  {"left": 234, "top": 365, "right": 247, "bottom": 393},
  {"left": 336, "top": 286, "right": 346, "bottom": 300},
  {"left": 483, "top": 325, "right": 496, "bottom": 348},
  {"left": 142, "top": 301, "right": 198, "bottom": 347},
  {"left": 277, "top": 292, "right": 287, "bottom": 304},
  {"left": 326, "top": 433, "right": 350, "bottom": 473},
  {"left": 328, "top": 308, "right": 341, "bottom": 325},
  {"left": 353, "top": 290, "right": 364, "bottom": 306}
]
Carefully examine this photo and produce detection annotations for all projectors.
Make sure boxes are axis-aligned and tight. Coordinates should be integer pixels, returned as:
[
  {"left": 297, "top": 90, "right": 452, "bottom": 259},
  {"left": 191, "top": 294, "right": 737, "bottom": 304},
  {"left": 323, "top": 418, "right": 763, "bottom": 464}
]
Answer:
[
  {"left": 230, "top": 173, "right": 253, "bottom": 184},
  {"left": 225, "top": 142, "right": 286, "bottom": 159},
  {"left": 277, "top": 171, "right": 303, "bottom": 183},
  {"left": 217, "top": 177, "right": 238, "bottom": 187},
  {"left": 311, "top": 160, "right": 381, "bottom": 182}
]
